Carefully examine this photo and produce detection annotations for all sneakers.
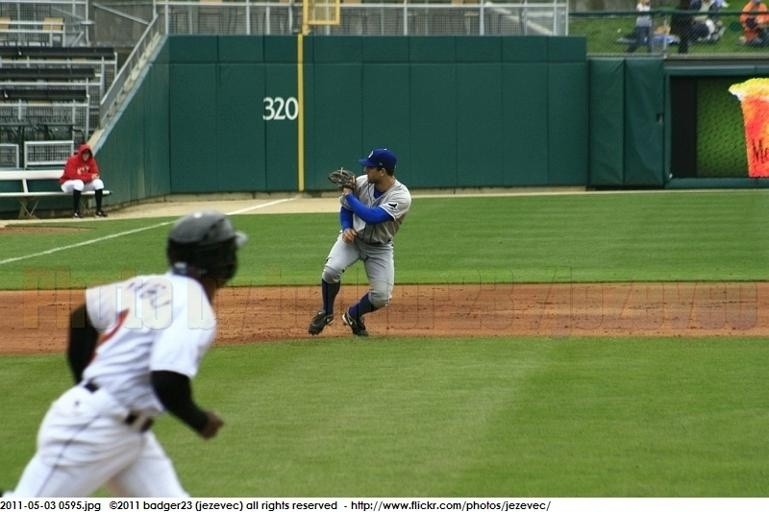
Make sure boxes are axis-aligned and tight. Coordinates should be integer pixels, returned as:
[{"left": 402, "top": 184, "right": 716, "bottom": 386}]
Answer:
[
  {"left": 72, "top": 209, "right": 109, "bottom": 218},
  {"left": 309, "top": 309, "right": 333, "bottom": 336},
  {"left": 342, "top": 309, "right": 368, "bottom": 336}
]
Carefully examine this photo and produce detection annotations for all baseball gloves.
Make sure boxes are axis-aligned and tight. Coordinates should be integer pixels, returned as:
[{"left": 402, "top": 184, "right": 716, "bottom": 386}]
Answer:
[{"left": 328, "top": 170, "right": 357, "bottom": 192}]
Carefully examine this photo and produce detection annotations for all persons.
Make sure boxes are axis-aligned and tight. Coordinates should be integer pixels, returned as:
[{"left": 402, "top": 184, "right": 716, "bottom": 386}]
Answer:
[
  {"left": 622, "top": 0, "right": 769, "bottom": 53},
  {"left": 308, "top": 148, "right": 411, "bottom": 337},
  {"left": 0, "top": 210, "right": 248, "bottom": 497},
  {"left": 58, "top": 144, "right": 107, "bottom": 218}
]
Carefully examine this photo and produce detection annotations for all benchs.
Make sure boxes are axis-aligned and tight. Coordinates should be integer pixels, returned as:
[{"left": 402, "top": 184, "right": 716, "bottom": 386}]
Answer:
[
  {"left": 0, "top": 170, "right": 112, "bottom": 217},
  {"left": 0, "top": 44, "right": 118, "bottom": 167}
]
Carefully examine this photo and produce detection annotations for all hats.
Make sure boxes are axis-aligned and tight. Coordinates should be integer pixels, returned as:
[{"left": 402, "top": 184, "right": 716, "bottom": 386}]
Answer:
[{"left": 358, "top": 148, "right": 397, "bottom": 170}]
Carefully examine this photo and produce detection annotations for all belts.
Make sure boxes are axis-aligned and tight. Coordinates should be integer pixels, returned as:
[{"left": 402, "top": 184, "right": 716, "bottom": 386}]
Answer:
[{"left": 78, "top": 377, "right": 155, "bottom": 438}]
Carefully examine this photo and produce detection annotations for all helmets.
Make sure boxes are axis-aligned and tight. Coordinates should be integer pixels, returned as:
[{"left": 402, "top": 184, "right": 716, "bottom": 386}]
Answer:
[{"left": 167, "top": 209, "right": 247, "bottom": 264}]
